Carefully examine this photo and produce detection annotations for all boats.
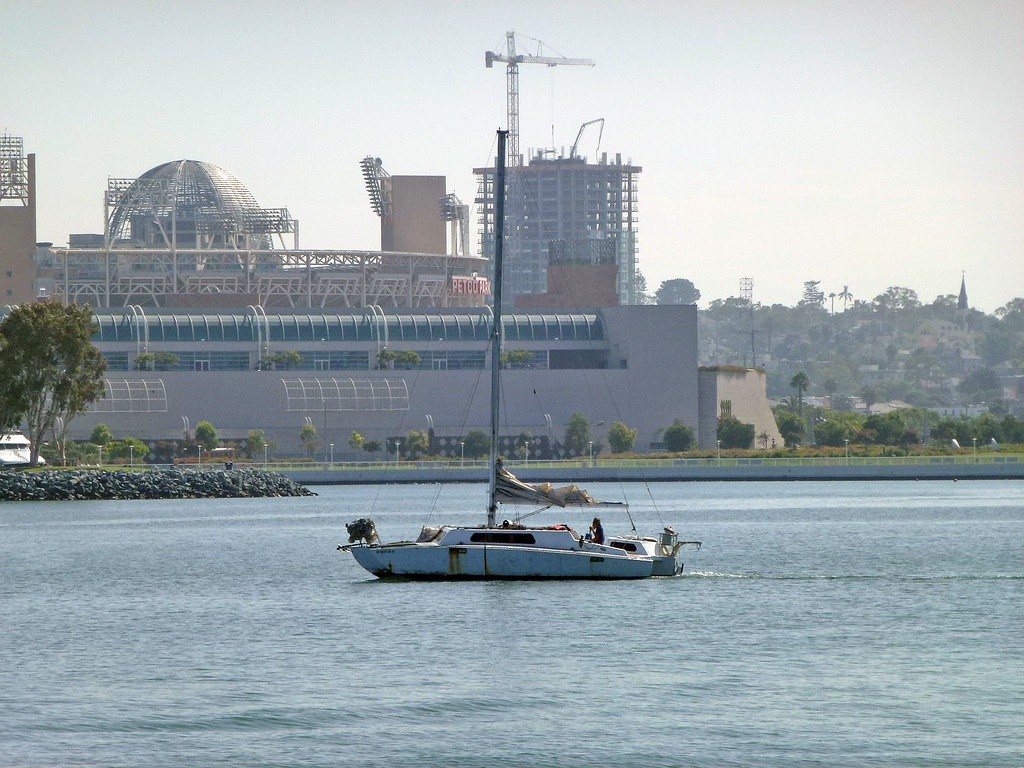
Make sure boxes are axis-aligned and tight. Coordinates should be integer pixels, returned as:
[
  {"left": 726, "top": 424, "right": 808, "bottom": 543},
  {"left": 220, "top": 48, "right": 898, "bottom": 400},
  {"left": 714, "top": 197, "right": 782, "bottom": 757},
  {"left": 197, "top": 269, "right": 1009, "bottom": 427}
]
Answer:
[
  {"left": 1, "top": 429, "right": 46, "bottom": 467},
  {"left": 337, "top": 126, "right": 707, "bottom": 579}
]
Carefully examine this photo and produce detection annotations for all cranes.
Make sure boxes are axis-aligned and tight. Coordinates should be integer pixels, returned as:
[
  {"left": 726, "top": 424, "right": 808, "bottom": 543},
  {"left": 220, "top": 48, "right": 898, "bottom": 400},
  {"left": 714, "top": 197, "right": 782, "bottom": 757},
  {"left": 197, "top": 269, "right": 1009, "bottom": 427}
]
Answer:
[{"left": 486, "top": 30, "right": 596, "bottom": 166}]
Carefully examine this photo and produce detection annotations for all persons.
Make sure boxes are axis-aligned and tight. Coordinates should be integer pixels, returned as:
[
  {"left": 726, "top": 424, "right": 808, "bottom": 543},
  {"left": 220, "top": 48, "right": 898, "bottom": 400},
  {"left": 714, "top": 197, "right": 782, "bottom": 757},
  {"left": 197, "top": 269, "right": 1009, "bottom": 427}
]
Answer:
[{"left": 589, "top": 518, "right": 606, "bottom": 546}]
